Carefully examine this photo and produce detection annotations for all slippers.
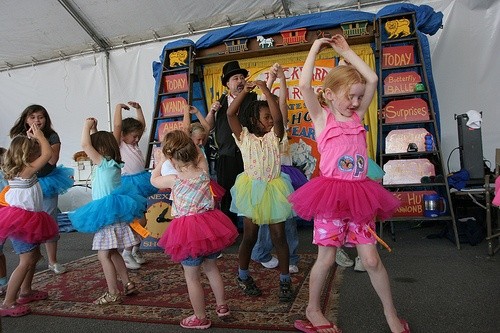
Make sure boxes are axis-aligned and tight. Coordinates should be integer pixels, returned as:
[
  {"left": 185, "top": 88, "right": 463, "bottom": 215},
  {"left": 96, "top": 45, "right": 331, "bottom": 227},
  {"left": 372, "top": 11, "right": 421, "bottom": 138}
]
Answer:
[
  {"left": 294, "top": 319, "right": 342, "bottom": 333},
  {"left": 400, "top": 319, "right": 410, "bottom": 333}
]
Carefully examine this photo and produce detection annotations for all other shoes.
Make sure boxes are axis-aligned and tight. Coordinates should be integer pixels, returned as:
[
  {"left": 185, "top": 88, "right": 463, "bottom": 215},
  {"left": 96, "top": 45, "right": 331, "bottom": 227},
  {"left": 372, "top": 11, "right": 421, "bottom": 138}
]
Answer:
[
  {"left": 48, "top": 263, "right": 65, "bottom": 275},
  {"left": 0, "top": 284, "right": 8, "bottom": 295}
]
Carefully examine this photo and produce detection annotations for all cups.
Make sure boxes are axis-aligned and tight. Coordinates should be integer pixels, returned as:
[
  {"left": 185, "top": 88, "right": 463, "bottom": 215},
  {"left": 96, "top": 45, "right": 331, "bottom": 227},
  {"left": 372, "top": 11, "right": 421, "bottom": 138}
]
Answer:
[{"left": 422, "top": 194, "right": 447, "bottom": 218}]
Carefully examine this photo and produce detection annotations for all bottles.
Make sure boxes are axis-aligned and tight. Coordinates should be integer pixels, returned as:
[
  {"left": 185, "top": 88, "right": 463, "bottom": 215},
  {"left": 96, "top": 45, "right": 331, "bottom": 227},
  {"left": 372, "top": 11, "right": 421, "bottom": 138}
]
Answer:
[{"left": 425, "top": 132, "right": 432, "bottom": 151}]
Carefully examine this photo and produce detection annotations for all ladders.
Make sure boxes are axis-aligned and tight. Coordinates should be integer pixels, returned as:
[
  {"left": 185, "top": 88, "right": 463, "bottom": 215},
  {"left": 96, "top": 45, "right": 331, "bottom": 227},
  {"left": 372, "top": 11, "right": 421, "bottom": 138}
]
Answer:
[
  {"left": 379, "top": 11, "right": 461, "bottom": 250},
  {"left": 144, "top": 39, "right": 195, "bottom": 172}
]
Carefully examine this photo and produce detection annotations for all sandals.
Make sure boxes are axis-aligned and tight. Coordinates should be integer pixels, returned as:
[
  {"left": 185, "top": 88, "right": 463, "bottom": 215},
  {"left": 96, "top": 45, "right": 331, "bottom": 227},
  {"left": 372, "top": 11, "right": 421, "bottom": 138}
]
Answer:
[
  {"left": 16, "top": 291, "right": 48, "bottom": 304},
  {"left": 124, "top": 280, "right": 137, "bottom": 296},
  {"left": 180, "top": 315, "right": 211, "bottom": 329},
  {"left": 216, "top": 304, "right": 232, "bottom": 320},
  {"left": 0, "top": 302, "right": 32, "bottom": 316},
  {"left": 93, "top": 291, "right": 123, "bottom": 306}
]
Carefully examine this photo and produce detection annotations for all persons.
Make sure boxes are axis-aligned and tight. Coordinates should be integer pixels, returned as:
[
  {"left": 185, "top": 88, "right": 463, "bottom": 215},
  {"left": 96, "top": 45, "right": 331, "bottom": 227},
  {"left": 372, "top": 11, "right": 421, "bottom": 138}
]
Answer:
[
  {"left": 251, "top": 63, "right": 309, "bottom": 273},
  {"left": 182, "top": 104, "right": 211, "bottom": 154},
  {"left": 113, "top": 101, "right": 159, "bottom": 268},
  {"left": 150, "top": 130, "right": 239, "bottom": 329},
  {"left": 67, "top": 118, "right": 148, "bottom": 304},
  {"left": 0, "top": 124, "right": 58, "bottom": 317},
  {"left": 10, "top": 105, "right": 75, "bottom": 274},
  {"left": 227, "top": 80, "right": 298, "bottom": 300},
  {"left": 205, "top": 62, "right": 263, "bottom": 257},
  {"left": 287, "top": 33, "right": 410, "bottom": 333}
]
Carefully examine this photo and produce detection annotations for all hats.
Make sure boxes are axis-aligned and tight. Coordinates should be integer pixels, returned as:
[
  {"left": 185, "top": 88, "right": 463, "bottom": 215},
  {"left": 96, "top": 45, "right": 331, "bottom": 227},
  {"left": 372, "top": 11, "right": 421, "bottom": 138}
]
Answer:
[{"left": 222, "top": 61, "right": 248, "bottom": 86}]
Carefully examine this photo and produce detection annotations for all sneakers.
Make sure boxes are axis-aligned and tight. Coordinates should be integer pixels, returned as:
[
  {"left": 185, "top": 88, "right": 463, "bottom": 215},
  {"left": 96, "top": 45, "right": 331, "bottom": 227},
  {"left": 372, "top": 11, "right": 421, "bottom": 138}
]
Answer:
[
  {"left": 354, "top": 256, "right": 367, "bottom": 271},
  {"left": 261, "top": 256, "right": 279, "bottom": 268},
  {"left": 278, "top": 279, "right": 294, "bottom": 302},
  {"left": 289, "top": 264, "right": 299, "bottom": 274},
  {"left": 335, "top": 249, "right": 354, "bottom": 267},
  {"left": 237, "top": 272, "right": 262, "bottom": 298},
  {"left": 122, "top": 250, "right": 145, "bottom": 269}
]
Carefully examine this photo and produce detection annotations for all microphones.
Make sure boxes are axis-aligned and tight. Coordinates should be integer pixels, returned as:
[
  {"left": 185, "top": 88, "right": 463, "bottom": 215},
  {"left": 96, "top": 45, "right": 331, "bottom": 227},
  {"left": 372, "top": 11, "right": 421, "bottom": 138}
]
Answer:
[{"left": 212, "top": 90, "right": 228, "bottom": 113}]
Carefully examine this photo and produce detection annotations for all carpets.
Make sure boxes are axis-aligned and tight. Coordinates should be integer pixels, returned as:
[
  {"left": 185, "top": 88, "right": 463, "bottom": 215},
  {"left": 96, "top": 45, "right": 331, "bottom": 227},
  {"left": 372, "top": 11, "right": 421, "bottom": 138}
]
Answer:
[{"left": 0, "top": 252, "right": 344, "bottom": 333}]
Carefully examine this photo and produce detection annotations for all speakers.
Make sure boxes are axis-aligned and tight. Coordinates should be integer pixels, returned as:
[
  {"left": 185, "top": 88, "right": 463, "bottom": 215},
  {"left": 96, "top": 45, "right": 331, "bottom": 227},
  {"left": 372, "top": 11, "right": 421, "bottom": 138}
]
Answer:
[{"left": 457, "top": 114, "right": 483, "bottom": 179}]
